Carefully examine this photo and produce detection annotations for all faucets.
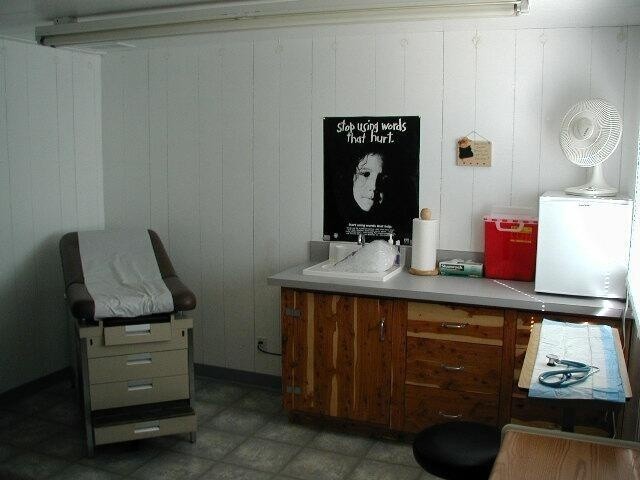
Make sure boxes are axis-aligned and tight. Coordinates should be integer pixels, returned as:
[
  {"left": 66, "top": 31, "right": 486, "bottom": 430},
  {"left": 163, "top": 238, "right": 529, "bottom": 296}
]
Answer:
[{"left": 357, "top": 232, "right": 366, "bottom": 248}]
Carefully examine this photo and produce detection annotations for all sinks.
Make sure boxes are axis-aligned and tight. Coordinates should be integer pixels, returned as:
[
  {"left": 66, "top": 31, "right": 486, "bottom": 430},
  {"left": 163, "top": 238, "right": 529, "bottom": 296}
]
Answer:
[{"left": 303, "top": 242, "right": 406, "bottom": 282}]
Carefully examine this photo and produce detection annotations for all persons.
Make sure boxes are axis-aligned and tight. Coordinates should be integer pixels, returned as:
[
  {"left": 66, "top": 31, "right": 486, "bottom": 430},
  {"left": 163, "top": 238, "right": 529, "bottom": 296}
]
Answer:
[{"left": 349, "top": 143, "right": 393, "bottom": 215}]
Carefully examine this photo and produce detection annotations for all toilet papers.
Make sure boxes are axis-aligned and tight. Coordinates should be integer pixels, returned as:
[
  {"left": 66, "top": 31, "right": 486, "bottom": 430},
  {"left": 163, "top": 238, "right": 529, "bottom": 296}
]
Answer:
[{"left": 410, "top": 219, "right": 439, "bottom": 270}]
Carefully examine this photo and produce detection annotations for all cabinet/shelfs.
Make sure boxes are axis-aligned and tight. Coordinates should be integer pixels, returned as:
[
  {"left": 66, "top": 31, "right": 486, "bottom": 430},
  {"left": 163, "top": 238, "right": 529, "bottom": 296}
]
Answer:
[{"left": 77, "top": 322, "right": 197, "bottom": 458}]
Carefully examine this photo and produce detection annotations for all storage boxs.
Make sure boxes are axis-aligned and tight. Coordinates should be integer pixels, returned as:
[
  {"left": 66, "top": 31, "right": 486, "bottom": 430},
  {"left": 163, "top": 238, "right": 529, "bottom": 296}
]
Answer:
[{"left": 481, "top": 206, "right": 538, "bottom": 282}]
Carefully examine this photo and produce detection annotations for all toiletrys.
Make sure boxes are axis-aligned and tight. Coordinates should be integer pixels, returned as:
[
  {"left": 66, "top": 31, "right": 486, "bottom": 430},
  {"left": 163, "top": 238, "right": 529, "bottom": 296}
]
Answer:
[{"left": 395, "top": 240, "right": 401, "bottom": 264}]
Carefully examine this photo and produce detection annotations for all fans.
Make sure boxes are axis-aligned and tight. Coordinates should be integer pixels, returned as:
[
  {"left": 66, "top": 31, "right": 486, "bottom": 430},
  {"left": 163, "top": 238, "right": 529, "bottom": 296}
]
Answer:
[{"left": 559, "top": 96, "right": 629, "bottom": 201}]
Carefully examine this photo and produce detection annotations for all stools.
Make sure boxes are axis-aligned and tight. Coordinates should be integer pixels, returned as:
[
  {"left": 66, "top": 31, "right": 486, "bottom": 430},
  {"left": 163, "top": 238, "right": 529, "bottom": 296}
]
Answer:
[{"left": 412, "top": 414, "right": 497, "bottom": 479}]
